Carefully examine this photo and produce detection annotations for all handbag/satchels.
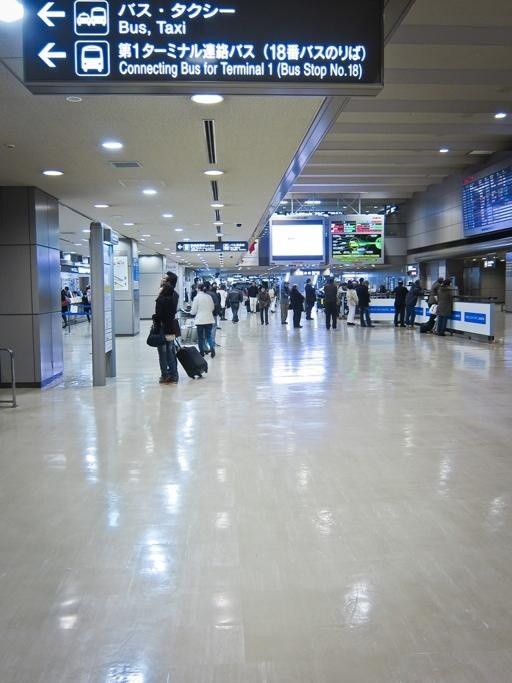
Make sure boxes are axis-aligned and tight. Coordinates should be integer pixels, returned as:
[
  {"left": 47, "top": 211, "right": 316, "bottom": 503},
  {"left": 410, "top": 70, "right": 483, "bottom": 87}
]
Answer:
[
  {"left": 420, "top": 320, "right": 436, "bottom": 333},
  {"left": 172, "top": 319, "right": 181, "bottom": 337},
  {"left": 428, "top": 304, "right": 439, "bottom": 316},
  {"left": 146, "top": 318, "right": 165, "bottom": 347},
  {"left": 62, "top": 296, "right": 70, "bottom": 307}
]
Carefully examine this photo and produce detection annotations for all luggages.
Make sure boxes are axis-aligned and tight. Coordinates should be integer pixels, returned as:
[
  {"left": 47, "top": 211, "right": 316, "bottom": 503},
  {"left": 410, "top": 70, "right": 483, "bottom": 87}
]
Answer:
[{"left": 173, "top": 338, "right": 208, "bottom": 379}]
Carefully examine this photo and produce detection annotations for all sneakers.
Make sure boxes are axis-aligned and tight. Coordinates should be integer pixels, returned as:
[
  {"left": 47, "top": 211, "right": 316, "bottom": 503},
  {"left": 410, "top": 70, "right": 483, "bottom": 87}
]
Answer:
[
  {"left": 395, "top": 324, "right": 399, "bottom": 327},
  {"left": 211, "top": 349, "right": 215, "bottom": 358},
  {"left": 216, "top": 310, "right": 376, "bottom": 329},
  {"left": 159, "top": 376, "right": 178, "bottom": 384},
  {"left": 400, "top": 324, "right": 406, "bottom": 327},
  {"left": 406, "top": 325, "right": 416, "bottom": 330}
]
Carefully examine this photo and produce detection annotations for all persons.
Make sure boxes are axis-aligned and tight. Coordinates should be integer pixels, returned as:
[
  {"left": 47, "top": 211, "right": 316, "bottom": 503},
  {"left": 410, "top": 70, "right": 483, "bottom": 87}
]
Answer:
[
  {"left": 184, "top": 278, "right": 279, "bottom": 325},
  {"left": 151, "top": 283, "right": 176, "bottom": 382},
  {"left": 61, "top": 285, "right": 90, "bottom": 328},
  {"left": 159, "top": 270, "right": 179, "bottom": 383},
  {"left": 203, "top": 281, "right": 220, "bottom": 354},
  {"left": 280, "top": 277, "right": 375, "bottom": 329},
  {"left": 188, "top": 283, "right": 216, "bottom": 358},
  {"left": 380, "top": 278, "right": 452, "bottom": 336}
]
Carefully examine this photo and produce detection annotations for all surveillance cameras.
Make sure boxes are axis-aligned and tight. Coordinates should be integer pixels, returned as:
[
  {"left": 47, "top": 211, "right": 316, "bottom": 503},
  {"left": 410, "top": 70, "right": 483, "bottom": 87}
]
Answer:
[{"left": 236, "top": 224, "right": 241, "bottom": 227}]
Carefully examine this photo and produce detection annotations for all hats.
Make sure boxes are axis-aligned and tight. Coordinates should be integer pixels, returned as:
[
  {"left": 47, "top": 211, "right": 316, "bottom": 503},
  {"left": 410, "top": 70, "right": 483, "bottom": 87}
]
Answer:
[{"left": 398, "top": 280, "right": 403, "bottom": 284}]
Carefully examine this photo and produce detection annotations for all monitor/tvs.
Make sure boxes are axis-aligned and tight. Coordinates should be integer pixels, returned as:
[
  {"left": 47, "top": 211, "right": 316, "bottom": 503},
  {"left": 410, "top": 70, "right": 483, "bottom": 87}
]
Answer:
[{"left": 406, "top": 264, "right": 418, "bottom": 272}]
